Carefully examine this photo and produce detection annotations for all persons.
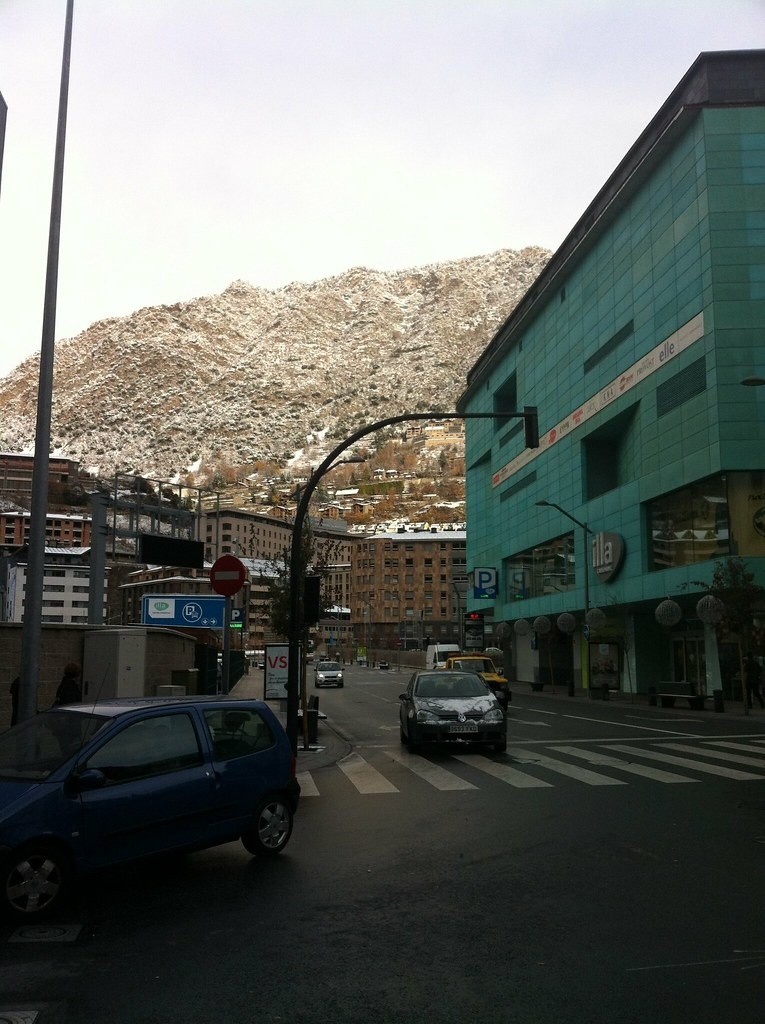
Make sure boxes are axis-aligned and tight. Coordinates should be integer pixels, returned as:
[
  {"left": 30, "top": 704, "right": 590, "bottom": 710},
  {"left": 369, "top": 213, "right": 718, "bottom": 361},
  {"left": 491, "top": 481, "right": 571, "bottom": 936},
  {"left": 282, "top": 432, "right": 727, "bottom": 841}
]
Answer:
[
  {"left": 9, "top": 676, "right": 21, "bottom": 728},
  {"left": 743, "top": 652, "right": 765, "bottom": 709},
  {"left": 51, "top": 662, "right": 82, "bottom": 707},
  {"left": 328, "top": 652, "right": 331, "bottom": 660},
  {"left": 119, "top": 727, "right": 176, "bottom": 783},
  {"left": 76, "top": 741, "right": 118, "bottom": 788},
  {"left": 335, "top": 651, "right": 340, "bottom": 662}
]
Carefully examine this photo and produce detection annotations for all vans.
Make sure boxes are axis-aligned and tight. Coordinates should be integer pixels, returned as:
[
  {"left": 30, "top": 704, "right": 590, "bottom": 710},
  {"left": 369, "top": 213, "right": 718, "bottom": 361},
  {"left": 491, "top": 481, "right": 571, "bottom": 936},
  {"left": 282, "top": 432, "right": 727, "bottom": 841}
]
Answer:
[{"left": 426, "top": 644, "right": 461, "bottom": 671}]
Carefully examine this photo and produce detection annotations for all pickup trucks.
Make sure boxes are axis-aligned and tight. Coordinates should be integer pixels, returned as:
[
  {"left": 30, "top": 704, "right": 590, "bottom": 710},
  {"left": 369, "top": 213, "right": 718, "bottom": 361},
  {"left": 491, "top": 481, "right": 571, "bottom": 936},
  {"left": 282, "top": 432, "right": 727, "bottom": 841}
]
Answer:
[{"left": 436, "top": 652, "right": 510, "bottom": 706}]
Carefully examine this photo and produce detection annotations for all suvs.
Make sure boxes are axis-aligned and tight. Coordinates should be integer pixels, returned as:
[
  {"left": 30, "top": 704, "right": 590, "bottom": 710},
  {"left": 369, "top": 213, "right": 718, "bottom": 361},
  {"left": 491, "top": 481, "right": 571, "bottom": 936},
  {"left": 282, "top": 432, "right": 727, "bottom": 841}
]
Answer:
[{"left": 1, "top": 695, "right": 302, "bottom": 925}]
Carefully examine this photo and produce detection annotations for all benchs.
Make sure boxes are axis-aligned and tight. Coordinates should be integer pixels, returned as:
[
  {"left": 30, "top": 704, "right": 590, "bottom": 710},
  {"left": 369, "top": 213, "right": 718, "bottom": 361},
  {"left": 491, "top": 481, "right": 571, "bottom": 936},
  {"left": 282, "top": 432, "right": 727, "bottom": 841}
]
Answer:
[
  {"left": 297, "top": 708, "right": 327, "bottom": 736},
  {"left": 657, "top": 692, "right": 704, "bottom": 710}
]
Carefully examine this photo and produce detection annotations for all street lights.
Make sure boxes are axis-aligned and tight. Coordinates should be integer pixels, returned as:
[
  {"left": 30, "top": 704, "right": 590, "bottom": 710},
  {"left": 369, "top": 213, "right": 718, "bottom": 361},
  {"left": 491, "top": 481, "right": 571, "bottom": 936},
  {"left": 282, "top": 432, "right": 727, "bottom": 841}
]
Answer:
[
  {"left": 449, "top": 582, "right": 463, "bottom": 645},
  {"left": 536, "top": 500, "right": 592, "bottom": 698}
]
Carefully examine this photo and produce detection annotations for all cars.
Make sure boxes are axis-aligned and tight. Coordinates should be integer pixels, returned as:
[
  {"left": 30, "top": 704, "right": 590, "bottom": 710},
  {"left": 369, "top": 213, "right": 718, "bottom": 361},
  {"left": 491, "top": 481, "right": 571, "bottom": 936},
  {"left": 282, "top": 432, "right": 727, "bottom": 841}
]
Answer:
[
  {"left": 399, "top": 669, "right": 508, "bottom": 754},
  {"left": 314, "top": 662, "right": 344, "bottom": 688}
]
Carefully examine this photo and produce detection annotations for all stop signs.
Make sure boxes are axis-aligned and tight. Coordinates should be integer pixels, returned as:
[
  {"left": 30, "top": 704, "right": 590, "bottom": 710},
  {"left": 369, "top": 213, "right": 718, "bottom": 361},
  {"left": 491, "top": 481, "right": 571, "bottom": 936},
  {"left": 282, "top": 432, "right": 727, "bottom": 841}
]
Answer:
[{"left": 210, "top": 556, "right": 245, "bottom": 597}]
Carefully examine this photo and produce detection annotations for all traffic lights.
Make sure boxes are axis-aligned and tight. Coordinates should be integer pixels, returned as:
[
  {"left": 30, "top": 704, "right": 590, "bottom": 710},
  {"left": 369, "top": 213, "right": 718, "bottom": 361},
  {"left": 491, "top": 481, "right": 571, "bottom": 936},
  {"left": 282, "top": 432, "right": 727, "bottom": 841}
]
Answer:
[{"left": 467, "top": 614, "right": 483, "bottom": 618}]
